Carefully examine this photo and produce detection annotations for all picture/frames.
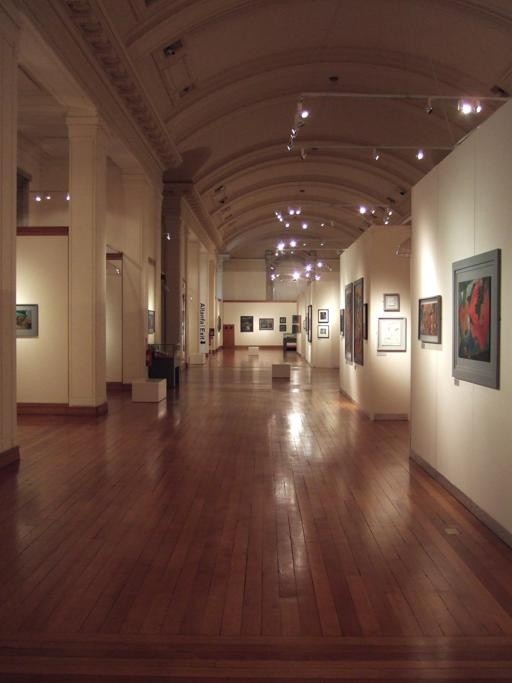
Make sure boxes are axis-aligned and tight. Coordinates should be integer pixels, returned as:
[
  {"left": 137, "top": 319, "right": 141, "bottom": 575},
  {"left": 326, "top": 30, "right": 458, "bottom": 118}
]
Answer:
[
  {"left": 382, "top": 292, "right": 401, "bottom": 312},
  {"left": 339, "top": 275, "right": 368, "bottom": 367},
  {"left": 307, "top": 304, "right": 312, "bottom": 342},
  {"left": 449, "top": 247, "right": 502, "bottom": 393},
  {"left": 279, "top": 314, "right": 300, "bottom": 333},
  {"left": 416, "top": 293, "right": 443, "bottom": 345},
  {"left": 15, "top": 302, "right": 39, "bottom": 338},
  {"left": 377, "top": 316, "right": 408, "bottom": 353},
  {"left": 259, "top": 318, "right": 274, "bottom": 330},
  {"left": 241, "top": 315, "right": 254, "bottom": 333},
  {"left": 316, "top": 308, "right": 330, "bottom": 338}
]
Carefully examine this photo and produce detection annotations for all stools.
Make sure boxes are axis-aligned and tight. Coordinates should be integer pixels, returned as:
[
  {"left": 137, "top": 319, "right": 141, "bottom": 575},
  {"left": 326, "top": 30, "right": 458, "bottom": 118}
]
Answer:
[
  {"left": 247, "top": 346, "right": 259, "bottom": 356},
  {"left": 272, "top": 362, "right": 292, "bottom": 378},
  {"left": 188, "top": 352, "right": 207, "bottom": 364},
  {"left": 131, "top": 378, "right": 168, "bottom": 403}
]
